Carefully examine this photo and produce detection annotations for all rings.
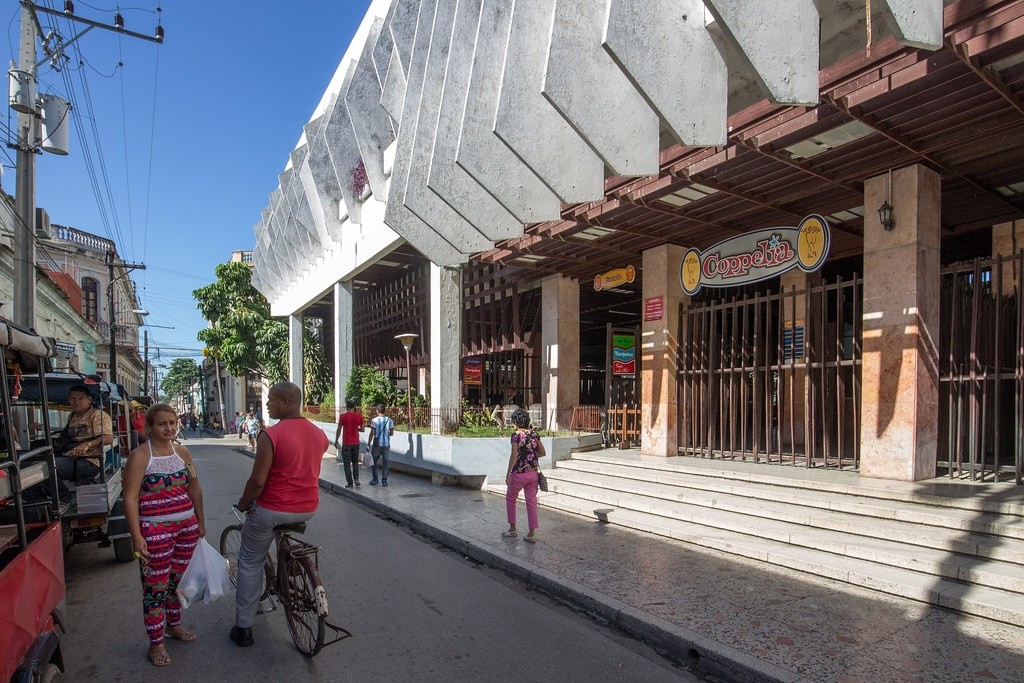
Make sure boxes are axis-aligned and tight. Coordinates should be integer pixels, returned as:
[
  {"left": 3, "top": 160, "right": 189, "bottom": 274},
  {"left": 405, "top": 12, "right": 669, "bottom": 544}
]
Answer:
[{"left": 135, "top": 552, "right": 141, "bottom": 559}]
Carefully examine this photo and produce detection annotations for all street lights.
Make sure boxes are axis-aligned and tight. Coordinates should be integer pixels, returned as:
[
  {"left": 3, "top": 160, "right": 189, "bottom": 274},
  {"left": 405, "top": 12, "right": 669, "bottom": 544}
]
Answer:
[
  {"left": 109, "top": 308, "right": 150, "bottom": 433},
  {"left": 394, "top": 334, "right": 420, "bottom": 431}
]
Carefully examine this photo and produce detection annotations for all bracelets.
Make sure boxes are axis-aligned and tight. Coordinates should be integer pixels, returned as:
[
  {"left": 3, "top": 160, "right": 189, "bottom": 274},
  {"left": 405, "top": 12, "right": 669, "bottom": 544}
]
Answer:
[{"left": 507, "top": 477, "right": 510, "bottom": 479}]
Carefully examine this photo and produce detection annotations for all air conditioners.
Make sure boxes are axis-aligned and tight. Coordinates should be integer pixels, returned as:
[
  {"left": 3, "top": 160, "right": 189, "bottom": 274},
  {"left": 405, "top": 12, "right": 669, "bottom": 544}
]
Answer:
[{"left": 35, "top": 206, "right": 52, "bottom": 240}]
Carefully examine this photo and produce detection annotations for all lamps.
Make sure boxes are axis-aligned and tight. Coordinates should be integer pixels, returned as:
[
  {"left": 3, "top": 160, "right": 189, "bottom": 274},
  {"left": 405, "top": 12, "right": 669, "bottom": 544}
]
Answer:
[{"left": 877, "top": 200, "right": 896, "bottom": 231}]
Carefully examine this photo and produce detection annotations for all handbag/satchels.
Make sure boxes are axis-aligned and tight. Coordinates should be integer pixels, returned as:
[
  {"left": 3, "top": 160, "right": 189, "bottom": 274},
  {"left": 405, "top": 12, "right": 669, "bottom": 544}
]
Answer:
[
  {"left": 539, "top": 472, "right": 548, "bottom": 493},
  {"left": 373, "top": 437, "right": 379, "bottom": 448},
  {"left": 363, "top": 449, "right": 375, "bottom": 468},
  {"left": 176, "top": 536, "right": 231, "bottom": 611}
]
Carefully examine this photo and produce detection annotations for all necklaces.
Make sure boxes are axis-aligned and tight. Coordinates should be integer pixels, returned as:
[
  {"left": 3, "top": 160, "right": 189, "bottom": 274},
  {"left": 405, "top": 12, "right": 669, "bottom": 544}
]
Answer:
[{"left": 151, "top": 445, "right": 161, "bottom": 457}]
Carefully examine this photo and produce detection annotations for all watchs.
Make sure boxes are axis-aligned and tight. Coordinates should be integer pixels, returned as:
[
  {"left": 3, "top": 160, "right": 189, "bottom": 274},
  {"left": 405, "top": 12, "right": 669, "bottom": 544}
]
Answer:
[{"left": 84, "top": 440, "right": 92, "bottom": 449}]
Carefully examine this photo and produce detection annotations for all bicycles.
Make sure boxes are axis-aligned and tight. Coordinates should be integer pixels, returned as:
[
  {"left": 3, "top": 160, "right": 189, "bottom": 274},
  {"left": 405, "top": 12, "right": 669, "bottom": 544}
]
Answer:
[{"left": 218, "top": 502, "right": 354, "bottom": 658}]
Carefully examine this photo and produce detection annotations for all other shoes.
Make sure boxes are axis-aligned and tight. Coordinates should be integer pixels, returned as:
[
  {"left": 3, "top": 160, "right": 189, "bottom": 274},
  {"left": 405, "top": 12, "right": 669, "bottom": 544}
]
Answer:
[
  {"left": 369, "top": 479, "right": 379, "bottom": 485},
  {"left": 523, "top": 534, "right": 536, "bottom": 543},
  {"left": 251, "top": 448, "right": 255, "bottom": 453},
  {"left": 502, "top": 529, "right": 518, "bottom": 538},
  {"left": 382, "top": 479, "right": 388, "bottom": 486},
  {"left": 354, "top": 477, "right": 361, "bottom": 486},
  {"left": 345, "top": 484, "right": 353, "bottom": 488}
]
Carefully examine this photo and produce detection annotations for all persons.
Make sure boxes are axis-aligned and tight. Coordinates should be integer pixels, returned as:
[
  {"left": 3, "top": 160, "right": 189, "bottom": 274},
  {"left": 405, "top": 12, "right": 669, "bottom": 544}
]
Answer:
[
  {"left": 230, "top": 381, "right": 329, "bottom": 645},
  {"left": 366, "top": 404, "right": 394, "bottom": 486},
  {"left": 116, "top": 402, "right": 148, "bottom": 455},
  {"left": 334, "top": 401, "right": 365, "bottom": 488},
  {"left": 174, "top": 410, "right": 220, "bottom": 440},
  {"left": 21, "top": 381, "right": 113, "bottom": 514},
  {"left": 502, "top": 408, "right": 546, "bottom": 543},
  {"left": 122, "top": 404, "right": 206, "bottom": 666},
  {"left": 234, "top": 407, "right": 261, "bottom": 452}
]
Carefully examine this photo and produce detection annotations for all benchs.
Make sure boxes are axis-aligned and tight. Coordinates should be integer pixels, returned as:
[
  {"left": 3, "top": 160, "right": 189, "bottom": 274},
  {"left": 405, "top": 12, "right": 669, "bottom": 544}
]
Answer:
[{"left": 29, "top": 436, "right": 121, "bottom": 485}]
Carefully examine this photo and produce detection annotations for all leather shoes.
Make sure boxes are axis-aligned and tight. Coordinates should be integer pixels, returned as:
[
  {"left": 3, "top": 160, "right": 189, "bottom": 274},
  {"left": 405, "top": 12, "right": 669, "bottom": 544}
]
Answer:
[{"left": 229, "top": 624, "right": 255, "bottom": 648}]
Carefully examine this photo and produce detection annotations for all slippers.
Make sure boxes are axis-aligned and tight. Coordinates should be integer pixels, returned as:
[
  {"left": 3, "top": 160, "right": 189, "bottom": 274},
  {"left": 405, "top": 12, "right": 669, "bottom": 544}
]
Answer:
[
  {"left": 164, "top": 629, "right": 198, "bottom": 642},
  {"left": 147, "top": 647, "right": 172, "bottom": 666}
]
